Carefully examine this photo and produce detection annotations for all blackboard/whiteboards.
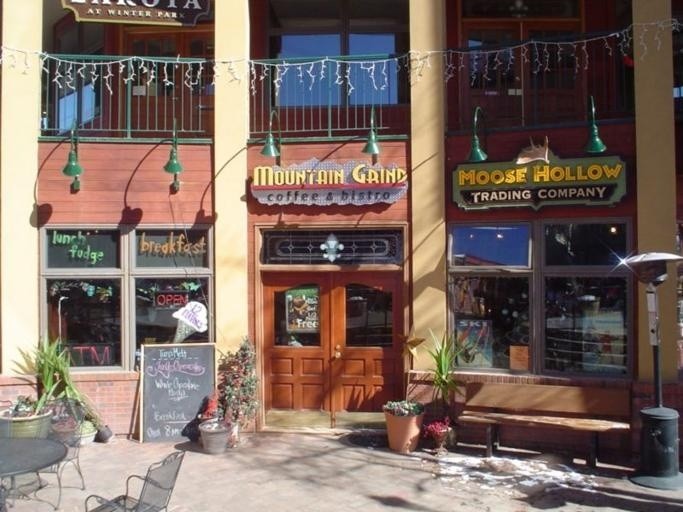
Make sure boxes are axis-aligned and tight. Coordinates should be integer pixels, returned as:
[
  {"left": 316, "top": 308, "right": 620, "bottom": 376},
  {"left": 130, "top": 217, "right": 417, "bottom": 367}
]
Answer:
[{"left": 139, "top": 342, "right": 218, "bottom": 444}]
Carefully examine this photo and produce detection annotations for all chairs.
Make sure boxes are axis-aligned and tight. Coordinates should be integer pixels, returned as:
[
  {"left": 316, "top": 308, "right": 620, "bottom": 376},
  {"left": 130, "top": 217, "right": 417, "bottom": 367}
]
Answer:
[{"left": 0, "top": 398, "right": 189, "bottom": 512}]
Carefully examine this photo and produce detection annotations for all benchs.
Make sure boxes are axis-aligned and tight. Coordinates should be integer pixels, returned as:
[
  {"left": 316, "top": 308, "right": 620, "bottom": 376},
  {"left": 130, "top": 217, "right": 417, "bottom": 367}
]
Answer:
[{"left": 461, "top": 401, "right": 631, "bottom": 470}]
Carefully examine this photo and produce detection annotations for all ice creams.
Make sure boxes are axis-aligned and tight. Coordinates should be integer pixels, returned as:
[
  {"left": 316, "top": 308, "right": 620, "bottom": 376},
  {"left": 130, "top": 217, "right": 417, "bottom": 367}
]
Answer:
[{"left": 172, "top": 301, "right": 208, "bottom": 343}]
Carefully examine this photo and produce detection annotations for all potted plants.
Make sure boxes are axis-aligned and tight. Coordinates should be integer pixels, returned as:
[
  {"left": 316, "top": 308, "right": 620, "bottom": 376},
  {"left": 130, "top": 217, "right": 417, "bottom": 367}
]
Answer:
[
  {"left": 195, "top": 341, "right": 262, "bottom": 454},
  {"left": 0, "top": 340, "right": 113, "bottom": 447},
  {"left": 382, "top": 328, "right": 462, "bottom": 454}
]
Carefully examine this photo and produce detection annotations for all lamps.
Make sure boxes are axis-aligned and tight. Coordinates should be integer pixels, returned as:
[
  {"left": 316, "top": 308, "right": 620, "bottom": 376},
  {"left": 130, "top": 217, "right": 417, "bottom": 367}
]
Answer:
[
  {"left": 260, "top": 108, "right": 281, "bottom": 157},
  {"left": 362, "top": 101, "right": 380, "bottom": 154},
  {"left": 164, "top": 118, "right": 182, "bottom": 192},
  {"left": 62, "top": 116, "right": 83, "bottom": 191},
  {"left": 582, "top": 99, "right": 608, "bottom": 154},
  {"left": 466, "top": 105, "right": 489, "bottom": 162}
]
